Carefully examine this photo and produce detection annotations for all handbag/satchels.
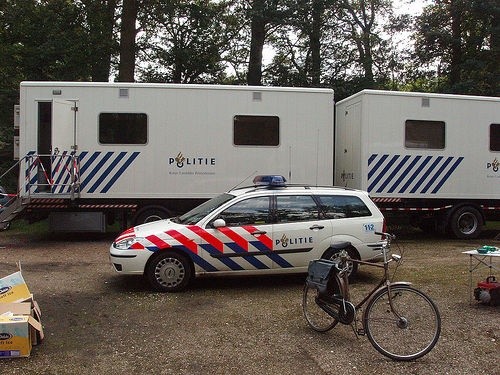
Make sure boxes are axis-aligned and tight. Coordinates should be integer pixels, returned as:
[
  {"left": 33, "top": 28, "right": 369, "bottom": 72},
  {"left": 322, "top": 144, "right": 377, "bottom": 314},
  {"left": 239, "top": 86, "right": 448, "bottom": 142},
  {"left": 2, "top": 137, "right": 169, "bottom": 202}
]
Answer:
[{"left": 307, "top": 258, "right": 337, "bottom": 296}]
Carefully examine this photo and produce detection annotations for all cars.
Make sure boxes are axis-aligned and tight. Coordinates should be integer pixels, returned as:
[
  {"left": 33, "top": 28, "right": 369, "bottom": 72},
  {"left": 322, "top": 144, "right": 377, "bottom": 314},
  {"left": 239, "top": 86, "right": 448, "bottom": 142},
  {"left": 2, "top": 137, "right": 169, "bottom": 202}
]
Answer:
[{"left": 111, "top": 174, "right": 391, "bottom": 292}]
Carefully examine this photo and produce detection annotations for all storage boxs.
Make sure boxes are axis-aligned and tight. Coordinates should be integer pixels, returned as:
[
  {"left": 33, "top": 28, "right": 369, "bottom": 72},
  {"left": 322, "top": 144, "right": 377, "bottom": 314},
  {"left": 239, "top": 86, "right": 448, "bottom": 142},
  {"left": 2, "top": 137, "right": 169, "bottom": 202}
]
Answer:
[{"left": 0, "top": 260, "right": 44, "bottom": 358}]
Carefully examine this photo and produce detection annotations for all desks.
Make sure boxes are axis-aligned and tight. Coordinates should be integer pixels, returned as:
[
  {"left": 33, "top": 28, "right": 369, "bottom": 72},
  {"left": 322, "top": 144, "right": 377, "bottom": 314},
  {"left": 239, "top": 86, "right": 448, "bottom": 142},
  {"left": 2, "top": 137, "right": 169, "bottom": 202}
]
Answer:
[{"left": 462, "top": 249, "right": 500, "bottom": 305}]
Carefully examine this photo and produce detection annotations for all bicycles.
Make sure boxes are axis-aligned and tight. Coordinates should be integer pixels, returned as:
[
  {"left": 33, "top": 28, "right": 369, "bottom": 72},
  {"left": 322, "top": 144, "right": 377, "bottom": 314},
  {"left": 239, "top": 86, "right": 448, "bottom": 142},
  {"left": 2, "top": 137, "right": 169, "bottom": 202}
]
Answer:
[{"left": 300, "top": 232, "right": 443, "bottom": 361}]
